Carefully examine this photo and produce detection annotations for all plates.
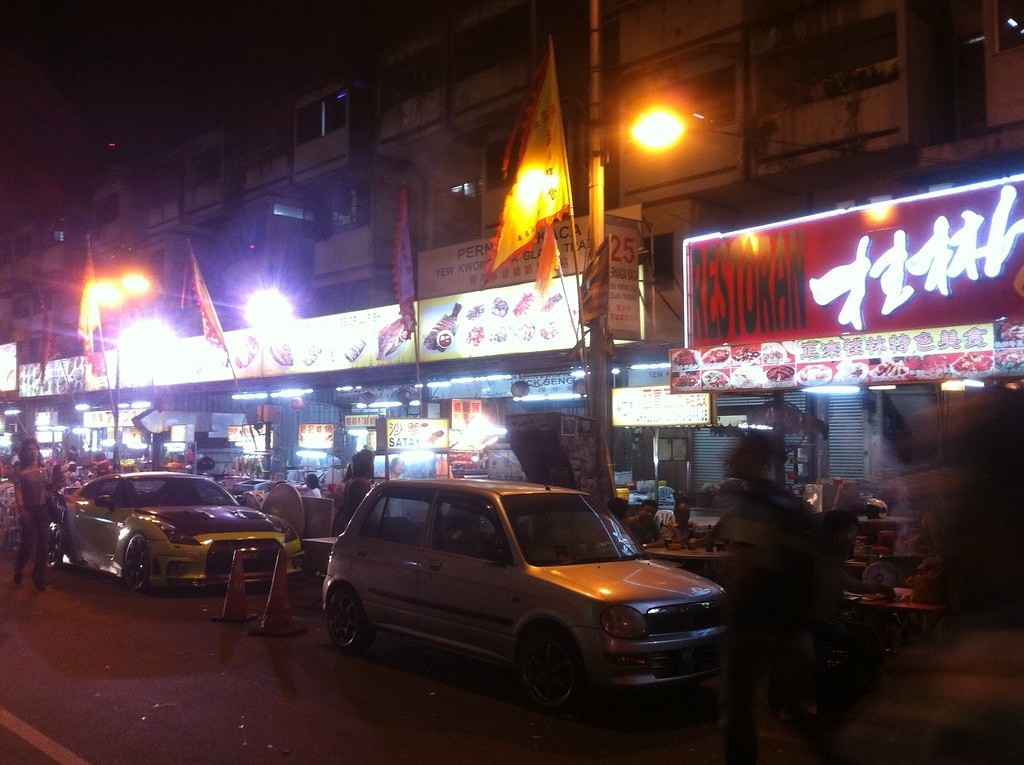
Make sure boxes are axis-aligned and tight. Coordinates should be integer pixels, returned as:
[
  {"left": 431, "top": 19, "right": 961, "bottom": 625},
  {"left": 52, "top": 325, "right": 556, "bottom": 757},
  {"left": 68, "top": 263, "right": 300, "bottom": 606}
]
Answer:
[{"left": 671, "top": 343, "right": 869, "bottom": 385}]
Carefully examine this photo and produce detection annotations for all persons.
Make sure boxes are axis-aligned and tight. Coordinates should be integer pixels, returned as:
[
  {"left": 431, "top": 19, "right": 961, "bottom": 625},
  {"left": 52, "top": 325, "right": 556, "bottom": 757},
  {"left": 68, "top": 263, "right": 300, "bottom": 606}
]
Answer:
[
  {"left": 52, "top": 449, "right": 83, "bottom": 567},
  {"left": 301, "top": 473, "right": 322, "bottom": 498},
  {"left": 712, "top": 429, "right": 898, "bottom": 765},
  {"left": 343, "top": 450, "right": 376, "bottom": 526},
  {"left": 660, "top": 500, "right": 711, "bottom": 548},
  {"left": 380, "top": 457, "right": 404, "bottom": 517},
  {"left": 9, "top": 445, "right": 21, "bottom": 466},
  {"left": 449, "top": 507, "right": 481, "bottom": 550},
  {"left": 627, "top": 500, "right": 665, "bottom": 548},
  {"left": 12, "top": 437, "right": 49, "bottom": 592},
  {"left": 232, "top": 447, "right": 262, "bottom": 476},
  {"left": 91, "top": 450, "right": 106, "bottom": 470},
  {"left": 607, "top": 497, "right": 629, "bottom": 523}
]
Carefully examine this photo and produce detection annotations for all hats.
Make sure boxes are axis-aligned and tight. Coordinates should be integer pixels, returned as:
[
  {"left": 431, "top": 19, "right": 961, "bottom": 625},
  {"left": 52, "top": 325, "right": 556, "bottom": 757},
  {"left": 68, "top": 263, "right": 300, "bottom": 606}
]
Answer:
[{"left": 307, "top": 475, "right": 318, "bottom": 485}]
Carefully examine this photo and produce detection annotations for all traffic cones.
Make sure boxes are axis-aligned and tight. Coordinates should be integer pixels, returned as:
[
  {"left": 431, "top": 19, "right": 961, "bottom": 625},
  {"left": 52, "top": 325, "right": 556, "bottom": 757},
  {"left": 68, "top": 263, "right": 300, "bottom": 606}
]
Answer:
[
  {"left": 209, "top": 548, "right": 260, "bottom": 624},
  {"left": 251, "top": 547, "right": 310, "bottom": 636}
]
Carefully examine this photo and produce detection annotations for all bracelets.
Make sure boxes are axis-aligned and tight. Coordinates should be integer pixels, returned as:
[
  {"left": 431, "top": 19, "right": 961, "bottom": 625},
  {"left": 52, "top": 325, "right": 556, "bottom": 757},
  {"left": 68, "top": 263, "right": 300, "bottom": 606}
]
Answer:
[{"left": 642, "top": 544, "right": 647, "bottom": 547}]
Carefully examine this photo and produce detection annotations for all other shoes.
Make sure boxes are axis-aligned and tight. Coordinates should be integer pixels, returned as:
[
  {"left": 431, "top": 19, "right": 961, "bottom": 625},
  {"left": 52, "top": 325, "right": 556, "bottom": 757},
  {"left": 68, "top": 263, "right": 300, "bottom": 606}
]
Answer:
[
  {"left": 32, "top": 573, "right": 48, "bottom": 592},
  {"left": 13, "top": 561, "right": 24, "bottom": 585}
]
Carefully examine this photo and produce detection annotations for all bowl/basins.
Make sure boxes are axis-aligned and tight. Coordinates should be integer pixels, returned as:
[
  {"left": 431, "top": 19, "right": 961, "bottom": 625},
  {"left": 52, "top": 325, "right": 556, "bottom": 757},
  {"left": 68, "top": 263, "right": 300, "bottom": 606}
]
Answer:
[
  {"left": 668, "top": 543, "right": 681, "bottom": 550},
  {"left": 893, "top": 587, "right": 913, "bottom": 599}
]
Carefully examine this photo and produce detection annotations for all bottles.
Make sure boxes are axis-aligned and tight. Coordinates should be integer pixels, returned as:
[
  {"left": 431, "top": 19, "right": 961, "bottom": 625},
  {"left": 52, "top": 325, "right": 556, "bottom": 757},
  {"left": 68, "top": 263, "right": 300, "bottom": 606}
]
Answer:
[
  {"left": 854, "top": 539, "right": 863, "bottom": 556},
  {"left": 687, "top": 524, "right": 696, "bottom": 549},
  {"left": 705, "top": 525, "right": 713, "bottom": 552}
]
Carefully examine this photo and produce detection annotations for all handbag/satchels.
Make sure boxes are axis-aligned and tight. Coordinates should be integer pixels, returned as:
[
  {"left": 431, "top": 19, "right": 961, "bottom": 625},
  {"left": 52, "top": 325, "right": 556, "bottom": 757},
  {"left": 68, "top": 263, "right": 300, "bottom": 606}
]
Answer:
[{"left": 49, "top": 484, "right": 67, "bottom": 523}]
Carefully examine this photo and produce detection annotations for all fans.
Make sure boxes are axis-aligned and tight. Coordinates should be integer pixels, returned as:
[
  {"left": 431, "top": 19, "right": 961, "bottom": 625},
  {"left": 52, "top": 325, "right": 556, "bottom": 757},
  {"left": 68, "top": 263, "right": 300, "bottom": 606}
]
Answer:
[{"left": 860, "top": 563, "right": 899, "bottom": 601}]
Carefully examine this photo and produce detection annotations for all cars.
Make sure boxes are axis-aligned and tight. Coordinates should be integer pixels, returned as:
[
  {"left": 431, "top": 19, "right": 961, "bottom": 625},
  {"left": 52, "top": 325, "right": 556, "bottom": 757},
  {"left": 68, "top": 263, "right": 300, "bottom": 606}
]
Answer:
[
  {"left": 60, "top": 470, "right": 308, "bottom": 594},
  {"left": 321, "top": 478, "right": 733, "bottom": 709}
]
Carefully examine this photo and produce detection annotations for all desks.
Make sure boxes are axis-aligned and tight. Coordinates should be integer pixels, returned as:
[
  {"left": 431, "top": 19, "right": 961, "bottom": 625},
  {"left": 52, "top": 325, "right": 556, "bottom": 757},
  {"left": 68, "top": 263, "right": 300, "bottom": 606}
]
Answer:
[
  {"left": 647, "top": 547, "right": 737, "bottom": 578},
  {"left": 850, "top": 597, "right": 943, "bottom": 653}
]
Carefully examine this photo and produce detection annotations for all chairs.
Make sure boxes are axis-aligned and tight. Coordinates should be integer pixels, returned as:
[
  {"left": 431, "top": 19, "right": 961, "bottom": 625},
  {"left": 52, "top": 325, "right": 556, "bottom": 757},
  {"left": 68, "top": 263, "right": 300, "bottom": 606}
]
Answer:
[{"left": 381, "top": 515, "right": 422, "bottom": 548}]
